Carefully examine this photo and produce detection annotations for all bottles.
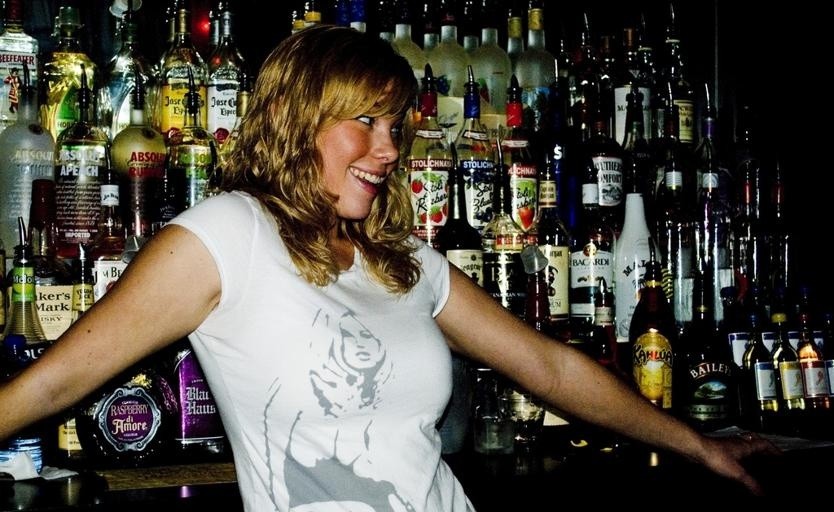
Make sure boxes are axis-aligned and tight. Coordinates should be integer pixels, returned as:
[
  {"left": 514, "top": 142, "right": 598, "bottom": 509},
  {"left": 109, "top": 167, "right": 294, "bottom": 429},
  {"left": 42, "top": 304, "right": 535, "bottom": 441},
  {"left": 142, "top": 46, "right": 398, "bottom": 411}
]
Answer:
[{"left": 0, "top": 0, "right": 834, "bottom": 475}]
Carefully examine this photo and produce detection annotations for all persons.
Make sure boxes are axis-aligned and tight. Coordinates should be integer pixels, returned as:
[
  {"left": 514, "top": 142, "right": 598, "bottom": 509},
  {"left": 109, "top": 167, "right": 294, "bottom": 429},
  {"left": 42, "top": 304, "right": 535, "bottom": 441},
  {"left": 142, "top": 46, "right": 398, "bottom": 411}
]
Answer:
[
  {"left": 263, "top": 300, "right": 419, "bottom": 512},
  {"left": 0, "top": 24, "right": 786, "bottom": 511}
]
